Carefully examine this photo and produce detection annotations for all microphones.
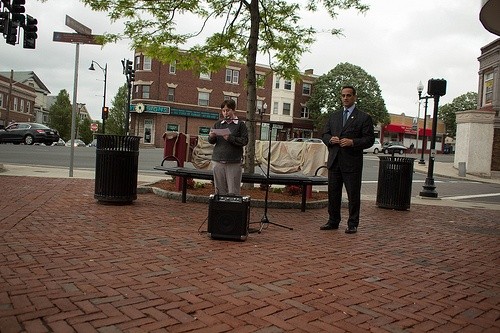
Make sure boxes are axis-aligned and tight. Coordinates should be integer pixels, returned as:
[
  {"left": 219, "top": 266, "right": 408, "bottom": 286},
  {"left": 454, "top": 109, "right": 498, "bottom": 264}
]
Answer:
[{"left": 223, "top": 117, "right": 230, "bottom": 120}]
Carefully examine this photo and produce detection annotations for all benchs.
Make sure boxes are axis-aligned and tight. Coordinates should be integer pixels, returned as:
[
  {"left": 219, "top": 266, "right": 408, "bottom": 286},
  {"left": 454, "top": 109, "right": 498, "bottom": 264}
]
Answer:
[{"left": 154, "top": 156, "right": 329, "bottom": 213}]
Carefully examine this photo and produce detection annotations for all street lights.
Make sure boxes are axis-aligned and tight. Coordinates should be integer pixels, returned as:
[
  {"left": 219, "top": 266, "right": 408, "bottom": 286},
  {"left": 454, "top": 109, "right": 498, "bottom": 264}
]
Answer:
[
  {"left": 257, "top": 102, "right": 267, "bottom": 141},
  {"left": 417, "top": 82, "right": 435, "bottom": 164},
  {"left": 88, "top": 60, "right": 107, "bottom": 134}
]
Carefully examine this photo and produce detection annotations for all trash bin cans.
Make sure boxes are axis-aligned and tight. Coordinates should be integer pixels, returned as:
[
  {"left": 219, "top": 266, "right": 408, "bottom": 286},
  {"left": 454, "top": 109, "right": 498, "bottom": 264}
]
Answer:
[
  {"left": 92, "top": 132, "right": 143, "bottom": 201},
  {"left": 375, "top": 156, "right": 417, "bottom": 209},
  {"left": 458, "top": 162, "right": 466, "bottom": 177}
]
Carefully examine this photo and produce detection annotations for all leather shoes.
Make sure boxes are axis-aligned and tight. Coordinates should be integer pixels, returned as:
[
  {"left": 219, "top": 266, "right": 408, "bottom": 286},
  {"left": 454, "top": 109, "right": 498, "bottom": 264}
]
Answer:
[
  {"left": 344, "top": 226, "right": 358, "bottom": 233},
  {"left": 320, "top": 223, "right": 338, "bottom": 229}
]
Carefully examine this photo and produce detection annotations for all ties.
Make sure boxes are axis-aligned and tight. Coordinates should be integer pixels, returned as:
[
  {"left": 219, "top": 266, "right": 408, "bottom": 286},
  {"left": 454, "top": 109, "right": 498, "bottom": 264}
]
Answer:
[{"left": 343, "top": 110, "right": 349, "bottom": 127}]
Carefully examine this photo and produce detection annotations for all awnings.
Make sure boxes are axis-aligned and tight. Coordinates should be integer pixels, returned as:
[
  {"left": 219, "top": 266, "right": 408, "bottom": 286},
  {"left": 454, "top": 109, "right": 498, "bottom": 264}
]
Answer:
[{"left": 382, "top": 123, "right": 432, "bottom": 136}]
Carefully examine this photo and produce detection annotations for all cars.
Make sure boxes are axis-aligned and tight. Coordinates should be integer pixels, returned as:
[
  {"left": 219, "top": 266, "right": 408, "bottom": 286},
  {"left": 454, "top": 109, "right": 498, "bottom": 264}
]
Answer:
[
  {"left": 65, "top": 139, "right": 86, "bottom": 148},
  {"left": 40, "top": 137, "right": 65, "bottom": 146},
  {"left": 382, "top": 141, "right": 408, "bottom": 154},
  {"left": 0, "top": 122, "right": 59, "bottom": 146},
  {"left": 87, "top": 139, "right": 97, "bottom": 147},
  {"left": 287, "top": 138, "right": 323, "bottom": 144}
]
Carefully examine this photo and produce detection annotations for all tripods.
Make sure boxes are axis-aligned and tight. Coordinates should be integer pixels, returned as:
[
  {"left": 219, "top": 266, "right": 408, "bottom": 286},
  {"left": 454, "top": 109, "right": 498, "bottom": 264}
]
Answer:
[{"left": 228, "top": 119, "right": 294, "bottom": 234}]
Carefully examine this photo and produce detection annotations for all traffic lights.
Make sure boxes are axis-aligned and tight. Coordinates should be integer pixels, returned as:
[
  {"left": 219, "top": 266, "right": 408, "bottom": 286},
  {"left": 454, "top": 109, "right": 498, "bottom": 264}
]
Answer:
[
  {"left": 126, "top": 60, "right": 132, "bottom": 74},
  {"left": 0, "top": 0, "right": 25, "bottom": 45},
  {"left": 130, "top": 69, "right": 135, "bottom": 81},
  {"left": 23, "top": 14, "right": 38, "bottom": 49},
  {"left": 102, "top": 107, "right": 108, "bottom": 119}
]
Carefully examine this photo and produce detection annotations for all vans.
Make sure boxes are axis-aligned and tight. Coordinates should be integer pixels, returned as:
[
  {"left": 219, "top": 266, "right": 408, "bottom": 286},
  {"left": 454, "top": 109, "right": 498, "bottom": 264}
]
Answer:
[{"left": 363, "top": 139, "right": 381, "bottom": 154}]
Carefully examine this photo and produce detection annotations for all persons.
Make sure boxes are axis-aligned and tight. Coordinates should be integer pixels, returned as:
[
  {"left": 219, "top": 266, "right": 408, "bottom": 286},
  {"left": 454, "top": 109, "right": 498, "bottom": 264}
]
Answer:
[
  {"left": 319, "top": 86, "right": 375, "bottom": 233},
  {"left": 208, "top": 99, "right": 248, "bottom": 196}
]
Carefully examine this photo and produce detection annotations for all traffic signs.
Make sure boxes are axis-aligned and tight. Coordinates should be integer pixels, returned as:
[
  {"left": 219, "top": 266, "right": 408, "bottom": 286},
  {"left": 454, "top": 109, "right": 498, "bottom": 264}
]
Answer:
[{"left": 53, "top": 15, "right": 105, "bottom": 44}]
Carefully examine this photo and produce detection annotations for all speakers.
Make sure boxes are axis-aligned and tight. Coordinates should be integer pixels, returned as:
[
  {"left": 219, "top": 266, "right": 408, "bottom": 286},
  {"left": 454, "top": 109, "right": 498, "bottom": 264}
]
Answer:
[{"left": 207, "top": 194, "right": 251, "bottom": 241}]
